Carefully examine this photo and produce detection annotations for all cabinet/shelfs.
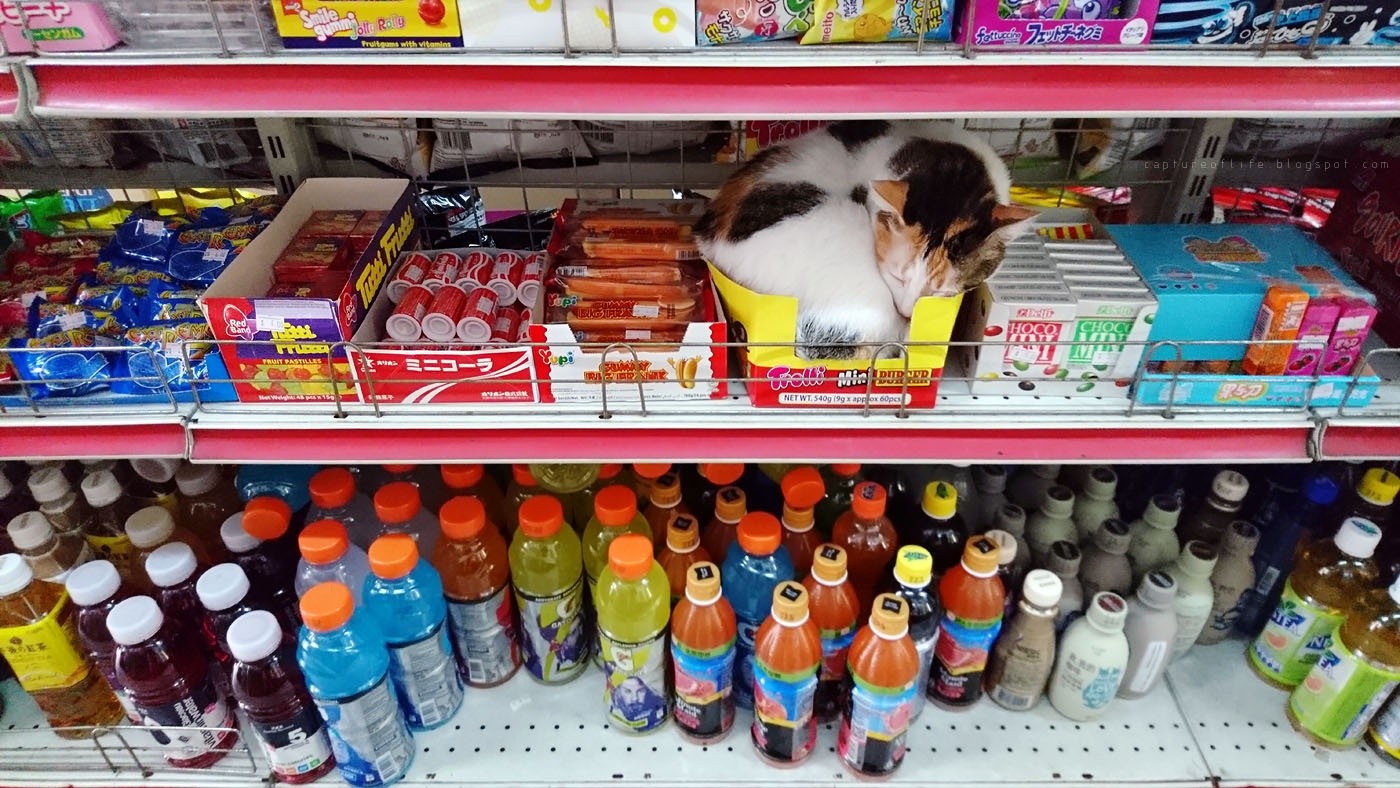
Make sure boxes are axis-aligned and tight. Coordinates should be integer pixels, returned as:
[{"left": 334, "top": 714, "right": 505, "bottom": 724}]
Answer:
[{"left": 0, "top": 46, "right": 1400, "bottom": 788}]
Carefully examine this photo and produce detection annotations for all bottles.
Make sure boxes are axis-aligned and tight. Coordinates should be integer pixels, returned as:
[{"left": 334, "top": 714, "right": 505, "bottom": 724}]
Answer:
[{"left": 0, "top": 458, "right": 1400, "bottom": 788}]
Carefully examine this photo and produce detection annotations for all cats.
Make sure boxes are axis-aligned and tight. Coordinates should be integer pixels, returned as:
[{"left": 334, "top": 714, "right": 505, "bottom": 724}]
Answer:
[{"left": 690, "top": 119, "right": 1013, "bottom": 360}]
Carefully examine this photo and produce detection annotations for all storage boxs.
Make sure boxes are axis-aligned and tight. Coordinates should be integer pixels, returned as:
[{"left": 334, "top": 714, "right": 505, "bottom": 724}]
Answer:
[
  {"left": 708, "top": 254, "right": 968, "bottom": 411},
  {"left": 0, "top": 0, "right": 1400, "bottom": 64},
  {"left": 971, "top": 237, "right": 1161, "bottom": 402},
  {"left": 195, "top": 175, "right": 424, "bottom": 403},
  {"left": 1105, "top": 222, "right": 1384, "bottom": 409},
  {"left": 525, "top": 200, "right": 728, "bottom": 406},
  {"left": 347, "top": 248, "right": 548, "bottom": 410},
  {"left": 0, "top": 347, "right": 239, "bottom": 408}
]
